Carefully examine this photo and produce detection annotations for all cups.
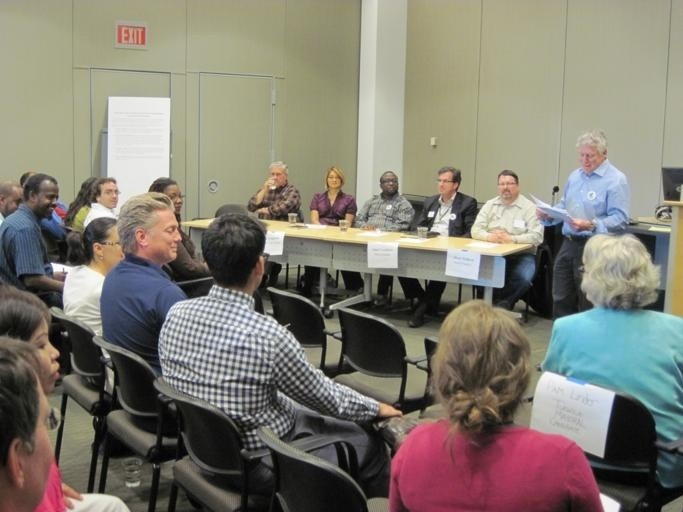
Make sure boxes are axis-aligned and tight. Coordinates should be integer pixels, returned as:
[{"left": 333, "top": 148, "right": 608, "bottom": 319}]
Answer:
[
  {"left": 339, "top": 220, "right": 349, "bottom": 233},
  {"left": 417, "top": 227, "right": 428, "bottom": 241},
  {"left": 121, "top": 458, "right": 143, "bottom": 489},
  {"left": 288, "top": 213, "right": 298, "bottom": 226},
  {"left": 269, "top": 179, "right": 276, "bottom": 190}
]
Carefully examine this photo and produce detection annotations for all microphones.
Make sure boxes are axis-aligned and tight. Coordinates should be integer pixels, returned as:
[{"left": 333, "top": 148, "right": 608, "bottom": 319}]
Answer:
[{"left": 552, "top": 186, "right": 559, "bottom": 195}]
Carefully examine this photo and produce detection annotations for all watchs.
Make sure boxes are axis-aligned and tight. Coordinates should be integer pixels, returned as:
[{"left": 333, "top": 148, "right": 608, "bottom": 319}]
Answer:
[
  {"left": 299, "top": 167, "right": 357, "bottom": 298},
  {"left": 470, "top": 169, "right": 546, "bottom": 313},
  {"left": 64, "top": 177, "right": 103, "bottom": 232},
  {"left": 589, "top": 220, "right": 594, "bottom": 231},
  {"left": 0, "top": 179, "right": 68, "bottom": 280},
  {"left": 398, "top": 166, "right": 479, "bottom": 327},
  {"left": 0, "top": 173, "right": 68, "bottom": 325},
  {"left": 83, "top": 177, "right": 122, "bottom": 230},
  {"left": 353, "top": 170, "right": 416, "bottom": 304},
  {"left": 388, "top": 298, "right": 604, "bottom": 512},
  {"left": 62, "top": 217, "right": 127, "bottom": 391},
  {"left": 148, "top": 177, "right": 213, "bottom": 284},
  {"left": 246, "top": 159, "right": 304, "bottom": 293},
  {"left": 99, "top": 190, "right": 192, "bottom": 439},
  {"left": 539, "top": 230, "right": 683, "bottom": 492},
  {"left": 20, "top": 171, "right": 67, "bottom": 241},
  {"left": 157, "top": 213, "right": 403, "bottom": 497},
  {"left": 0, "top": 332, "right": 53, "bottom": 512},
  {"left": 1, "top": 278, "right": 131, "bottom": 512},
  {"left": 535, "top": 126, "right": 630, "bottom": 318}
]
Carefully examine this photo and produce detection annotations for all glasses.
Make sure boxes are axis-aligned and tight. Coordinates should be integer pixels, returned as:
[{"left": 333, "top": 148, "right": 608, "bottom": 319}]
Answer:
[
  {"left": 435, "top": 178, "right": 457, "bottom": 184},
  {"left": 44, "top": 407, "right": 63, "bottom": 433},
  {"left": 577, "top": 266, "right": 584, "bottom": 273},
  {"left": 497, "top": 181, "right": 518, "bottom": 187},
  {"left": 92, "top": 239, "right": 121, "bottom": 247}
]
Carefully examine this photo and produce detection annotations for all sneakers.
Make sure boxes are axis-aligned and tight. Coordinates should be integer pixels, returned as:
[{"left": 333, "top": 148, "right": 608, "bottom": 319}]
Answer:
[
  {"left": 257, "top": 274, "right": 268, "bottom": 296},
  {"left": 373, "top": 294, "right": 392, "bottom": 306},
  {"left": 337, "top": 286, "right": 363, "bottom": 299},
  {"left": 268, "top": 262, "right": 282, "bottom": 286}
]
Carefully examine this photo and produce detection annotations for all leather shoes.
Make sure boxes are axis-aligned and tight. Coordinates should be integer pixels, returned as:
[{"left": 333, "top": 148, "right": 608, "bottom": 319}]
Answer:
[
  {"left": 408, "top": 304, "right": 425, "bottom": 327},
  {"left": 409, "top": 297, "right": 432, "bottom": 322}
]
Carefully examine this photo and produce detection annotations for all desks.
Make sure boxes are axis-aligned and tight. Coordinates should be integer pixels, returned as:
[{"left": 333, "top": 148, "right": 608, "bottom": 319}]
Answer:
[
  {"left": 181, "top": 218, "right": 291, "bottom": 253},
  {"left": 265, "top": 222, "right": 363, "bottom": 315},
  {"left": 324, "top": 229, "right": 533, "bottom": 327},
  {"left": 529, "top": 217, "right": 672, "bottom": 320}
]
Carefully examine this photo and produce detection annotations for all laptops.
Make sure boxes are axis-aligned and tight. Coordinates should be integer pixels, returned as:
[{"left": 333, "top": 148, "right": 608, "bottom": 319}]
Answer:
[{"left": 400, "top": 230, "right": 440, "bottom": 238}]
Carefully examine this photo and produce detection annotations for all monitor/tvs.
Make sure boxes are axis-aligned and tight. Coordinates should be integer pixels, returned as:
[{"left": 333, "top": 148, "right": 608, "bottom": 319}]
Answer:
[{"left": 662, "top": 166, "right": 682, "bottom": 201}]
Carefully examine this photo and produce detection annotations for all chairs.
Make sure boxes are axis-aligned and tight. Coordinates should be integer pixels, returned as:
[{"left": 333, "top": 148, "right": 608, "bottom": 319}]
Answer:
[
  {"left": 153, "top": 377, "right": 359, "bottom": 512},
  {"left": 285, "top": 263, "right": 289, "bottom": 289},
  {"left": 388, "top": 194, "right": 429, "bottom": 306},
  {"left": 92, "top": 336, "right": 189, "bottom": 512},
  {"left": 334, "top": 307, "right": 435, "bottom": 416},
  {"left": 162, "top": 263, "right": 214, "bottom": 289},
  {"left": 266, "top": 287, "right": 358, "bottom": 379},
  {"left": 215, "top": 204, "right": 249, "bottom": 216},
  {"left": 253, "top": 290, "right": 273, "bottom": 317},
  {"left": 48, "top": 306, "right": 123, "bottom": 493},
  {"left": 472, "top": 244, "right": 554, "bottom": 323},
  {"left": 296, "top": 263, "right": 339, "bottom": 291},
  {"left": 255, "top": 424, "right": 389, "bottom": 512},
  {"left": 409, "top": 202, "right": 485, "bottom": 309},
  {"left": 528, "top": 370, "right": 683, "bottom": 512},
  {"left": 402, "top": 336, "right": 533, "bottom": 427}
]
